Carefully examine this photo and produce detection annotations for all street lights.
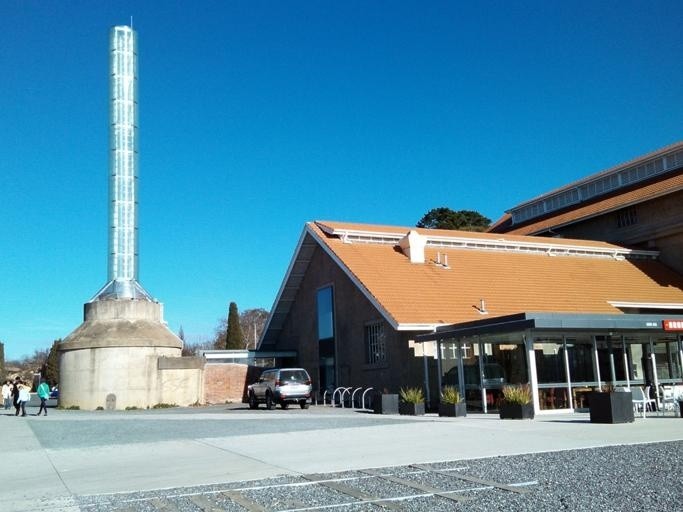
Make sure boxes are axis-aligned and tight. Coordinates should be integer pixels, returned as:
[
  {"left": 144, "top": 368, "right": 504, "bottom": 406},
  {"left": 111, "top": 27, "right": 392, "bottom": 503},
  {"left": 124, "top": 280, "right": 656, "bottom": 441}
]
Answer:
[
  {"left": 30, "top": 371, "right": 40, "bottom": 387},
  {"left": 252, "top": 313, "right": 261, "bottom": 350}
]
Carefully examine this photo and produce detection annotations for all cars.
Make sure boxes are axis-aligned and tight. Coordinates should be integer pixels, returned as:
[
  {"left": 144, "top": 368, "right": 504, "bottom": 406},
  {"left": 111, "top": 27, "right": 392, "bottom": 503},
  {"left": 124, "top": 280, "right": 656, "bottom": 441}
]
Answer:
[
  {"left": 49, "top": 384, "right": 58, "bottom": 399},
  {"left": 442, "top": 362, "right": 506, "bottom": 401}
]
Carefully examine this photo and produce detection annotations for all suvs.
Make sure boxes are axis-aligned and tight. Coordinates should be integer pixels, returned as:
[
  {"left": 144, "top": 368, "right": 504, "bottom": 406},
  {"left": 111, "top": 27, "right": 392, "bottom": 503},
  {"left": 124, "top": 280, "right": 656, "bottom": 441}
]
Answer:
[{"left": 246, "top": 369, "right": 312, "bottom": 410}]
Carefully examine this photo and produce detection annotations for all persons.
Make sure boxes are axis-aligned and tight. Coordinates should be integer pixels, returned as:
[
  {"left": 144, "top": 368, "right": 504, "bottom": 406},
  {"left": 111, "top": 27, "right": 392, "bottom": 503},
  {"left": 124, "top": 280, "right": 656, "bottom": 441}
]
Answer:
[
  {"left": 37, "top": 379, "right": 50, "bottom": 417},
  {"left": 2, "top": 377, "right": 32, "bottom": 417}
]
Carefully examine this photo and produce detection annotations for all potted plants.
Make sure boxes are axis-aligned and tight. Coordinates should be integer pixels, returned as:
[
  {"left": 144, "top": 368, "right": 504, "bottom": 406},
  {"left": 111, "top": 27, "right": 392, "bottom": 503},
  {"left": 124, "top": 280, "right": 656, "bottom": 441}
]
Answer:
[
  {"left": 500, "top": 384, "right": 534, "bottom": 419},
  {"left": 399, "top": 386, "right": 425, "bottom": 416},
  {"left": 439, "top": 385, "right": 467, "bottom": 417},
  {"left": 588, "top": 385, "right": 634, "bottom": 423}
]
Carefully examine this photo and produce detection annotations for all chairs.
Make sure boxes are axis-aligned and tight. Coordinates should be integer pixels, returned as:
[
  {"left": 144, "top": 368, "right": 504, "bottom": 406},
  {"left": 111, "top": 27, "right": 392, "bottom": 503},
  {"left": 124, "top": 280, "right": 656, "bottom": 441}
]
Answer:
[{"left": 614, "top": 386, "right": 680, "bottom": 419}]
